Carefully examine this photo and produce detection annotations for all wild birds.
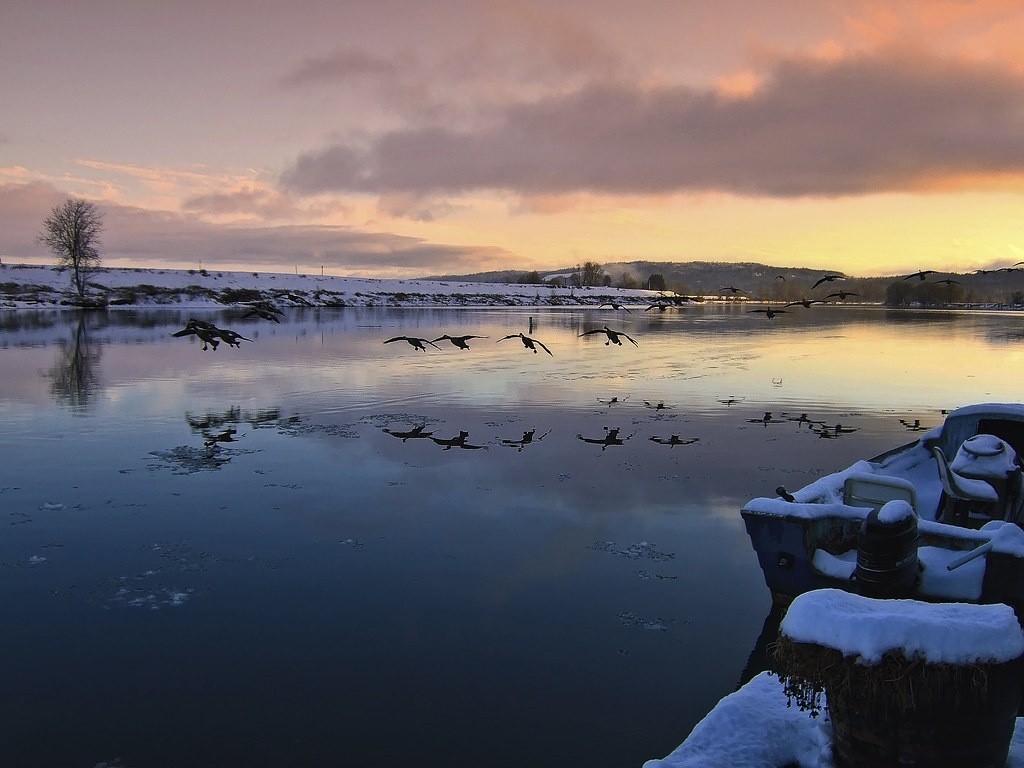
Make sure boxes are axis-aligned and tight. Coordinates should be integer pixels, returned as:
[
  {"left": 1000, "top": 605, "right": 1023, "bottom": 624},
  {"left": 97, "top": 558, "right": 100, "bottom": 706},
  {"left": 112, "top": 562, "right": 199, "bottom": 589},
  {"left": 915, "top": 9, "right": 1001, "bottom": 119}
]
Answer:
[
  {"left": 576, "top": 395, "right": 701, "bottom": 452},
  {"left": 576, "top": 295, "right": 701, "bottom": 348},
  {"left": 715, "top": 394, "right": 861, "bottom": 440},
  {"left": 168, "top": 317, "right": 254, "bottom": 351},
  {"left": 185, "top": 406, "right": 303, "bottom": 446},
  {"left": 719, "top": 274, "right": 864, "bottom": 320},
  {"left": 903, "top": 269, "right": 964, "bottom": 287},
  {"left": 381, "top": 423, "right": 551, "bottom": 453},
  {"left": 899, "top": 408, "right": 953, "bottom": 432},
  {"left": 383, "top": 332, "right": 554, "bottom": 357},
  {"left": 971, "top": 261, "right": 1024, "bottom": 275}
]
